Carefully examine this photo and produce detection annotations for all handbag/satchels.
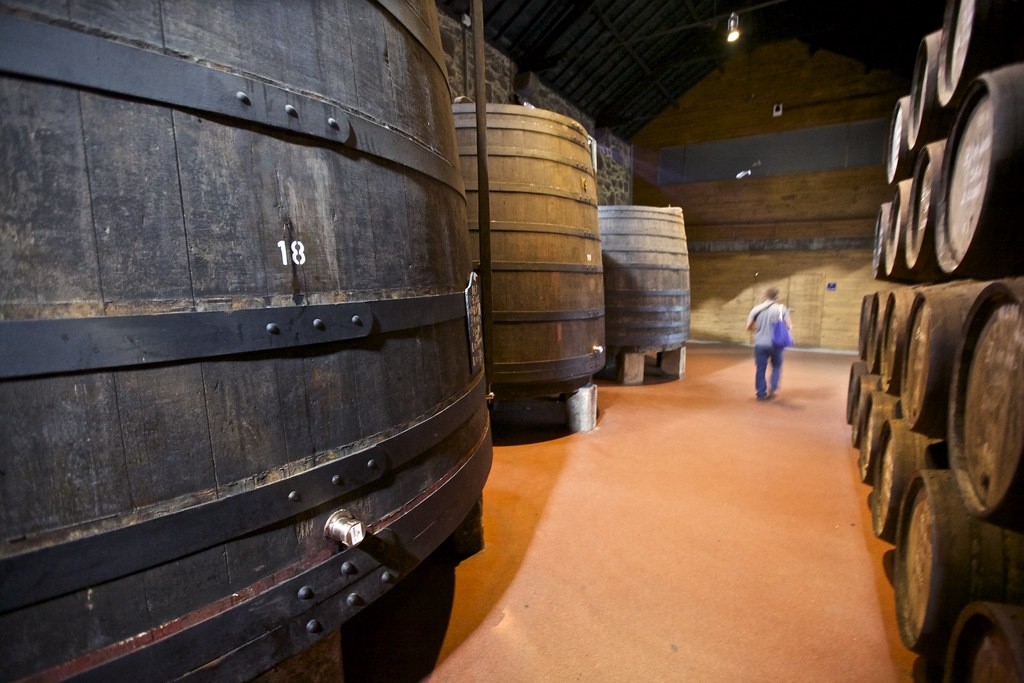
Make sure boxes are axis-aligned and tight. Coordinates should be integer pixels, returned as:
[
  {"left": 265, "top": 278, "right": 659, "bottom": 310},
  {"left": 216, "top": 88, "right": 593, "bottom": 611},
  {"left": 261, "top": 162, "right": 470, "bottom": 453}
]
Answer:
[{"left": 771, "top": 306, "right": 794, "bottom": 349}]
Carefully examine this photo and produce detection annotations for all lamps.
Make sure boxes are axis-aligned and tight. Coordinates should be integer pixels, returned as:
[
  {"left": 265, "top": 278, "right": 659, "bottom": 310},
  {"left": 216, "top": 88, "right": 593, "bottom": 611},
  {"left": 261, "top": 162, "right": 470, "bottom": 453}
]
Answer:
[{"left": 726, "top": 13, "right": 740, "bottom": 43}]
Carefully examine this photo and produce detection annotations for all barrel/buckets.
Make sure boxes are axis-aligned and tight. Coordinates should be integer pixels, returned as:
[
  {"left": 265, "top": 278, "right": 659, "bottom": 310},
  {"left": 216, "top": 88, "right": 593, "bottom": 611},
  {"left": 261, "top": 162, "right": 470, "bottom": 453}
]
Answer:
[
  {"left": 597, "top": 206, "right": 691, "bottom": 353},
  {"left": 0, "top": 0, "right": 494, "bottom": 683},
  {"left": 452, "top": 103, "right": 606, "bottom": 396},
  {"left": 847, "top": 0, "right": 1024, "bottom": 683}
]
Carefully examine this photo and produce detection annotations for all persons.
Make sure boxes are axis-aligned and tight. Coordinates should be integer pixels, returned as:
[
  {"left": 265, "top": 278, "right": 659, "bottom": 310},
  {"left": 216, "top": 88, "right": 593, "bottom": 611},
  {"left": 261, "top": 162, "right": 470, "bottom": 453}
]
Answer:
[{"left": 745, "top": 287, "right": 792, "bottom": 401}]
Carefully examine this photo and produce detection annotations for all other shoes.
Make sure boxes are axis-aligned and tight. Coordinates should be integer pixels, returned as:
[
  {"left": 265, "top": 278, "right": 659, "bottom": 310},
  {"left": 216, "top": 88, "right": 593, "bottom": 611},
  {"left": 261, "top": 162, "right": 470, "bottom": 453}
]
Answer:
[{"left": 758, "top": 393, "right": 776, "bottom": 400}]
